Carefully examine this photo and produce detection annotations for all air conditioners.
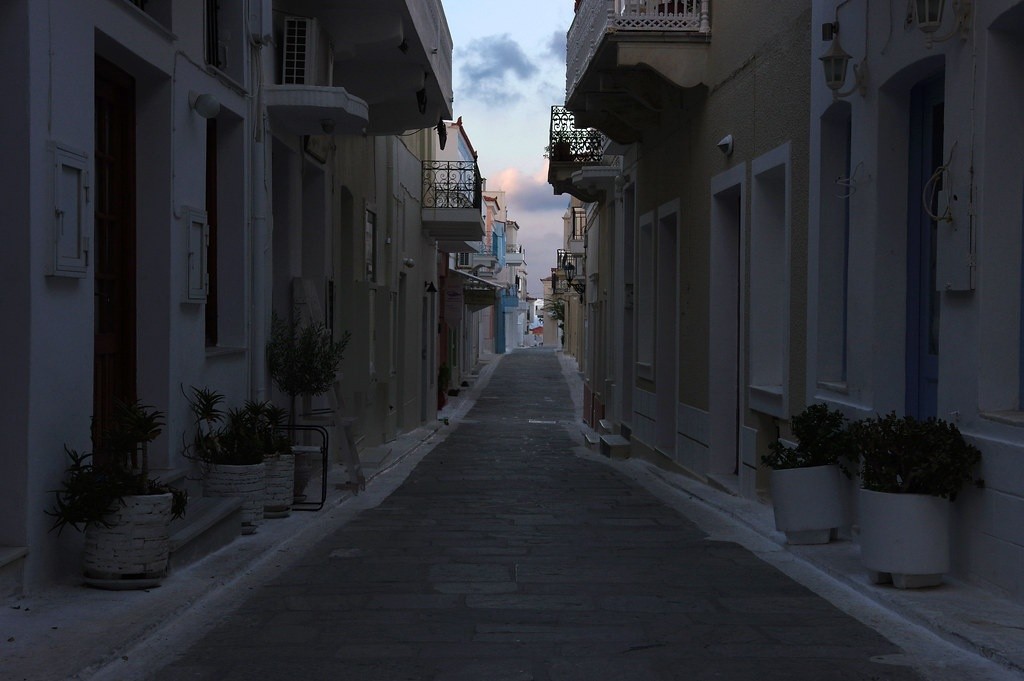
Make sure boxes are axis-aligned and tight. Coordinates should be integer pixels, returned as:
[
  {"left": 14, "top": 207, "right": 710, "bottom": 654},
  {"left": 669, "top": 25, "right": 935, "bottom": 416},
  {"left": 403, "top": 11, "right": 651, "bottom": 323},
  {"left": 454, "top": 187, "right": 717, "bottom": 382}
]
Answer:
[{"left": 280, "top": 15, "right": 330, "bottom": 84}]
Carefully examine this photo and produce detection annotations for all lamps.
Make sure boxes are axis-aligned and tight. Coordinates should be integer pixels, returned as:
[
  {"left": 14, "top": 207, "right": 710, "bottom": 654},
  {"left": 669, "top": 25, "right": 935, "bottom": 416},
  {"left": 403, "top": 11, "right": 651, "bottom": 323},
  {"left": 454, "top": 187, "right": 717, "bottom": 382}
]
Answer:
[
  {"left": 321, "top": 118, "right": 336, "bottom": 136},
  {"left": 404, "top": 256, "right": 418, "bottom": 269},
  {"left": 437, "top": 118, "right": 447, "bottom": 151},
  {"left": 187, "top": 90, "right": 221, "bottom": 119},
  {"left": 819, "top": 19, "right": 867, "bottom": 104},
  {"left": 417, "top": 87, "right": 428, "bottom": 116},
  {"left": 425, "top": 281, "right": 438, "bottom": 293},
  {"left": 564, "top": 261, "right": 585, "bottom": 295},
  {"left": 906, "top": 0, "right": 972, "bottom": 50}
]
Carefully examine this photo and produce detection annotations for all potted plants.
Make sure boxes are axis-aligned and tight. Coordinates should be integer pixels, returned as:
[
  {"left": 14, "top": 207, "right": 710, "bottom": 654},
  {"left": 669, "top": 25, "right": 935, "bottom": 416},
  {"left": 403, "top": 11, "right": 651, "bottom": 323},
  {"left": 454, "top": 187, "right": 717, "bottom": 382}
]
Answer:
[
  {"left": 179, "top": 278, "right": 353, "bottom": 534},
  {"left": 45, "top": 392, "right": 190, "bottom": 591},
  {"left": 759, "top": 400, "right": 985, "bottom": 590},
  {"left": 542, "top": 131, "right": 573, "bottom": 162}
]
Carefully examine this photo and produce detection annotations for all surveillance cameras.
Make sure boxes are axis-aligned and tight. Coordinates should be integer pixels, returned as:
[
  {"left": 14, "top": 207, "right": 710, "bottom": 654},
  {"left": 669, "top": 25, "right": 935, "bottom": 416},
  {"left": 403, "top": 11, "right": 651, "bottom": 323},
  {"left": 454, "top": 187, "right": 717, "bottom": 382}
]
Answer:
[{"left": 322, "top": 119, "right": 336, "bottom": 134}]
